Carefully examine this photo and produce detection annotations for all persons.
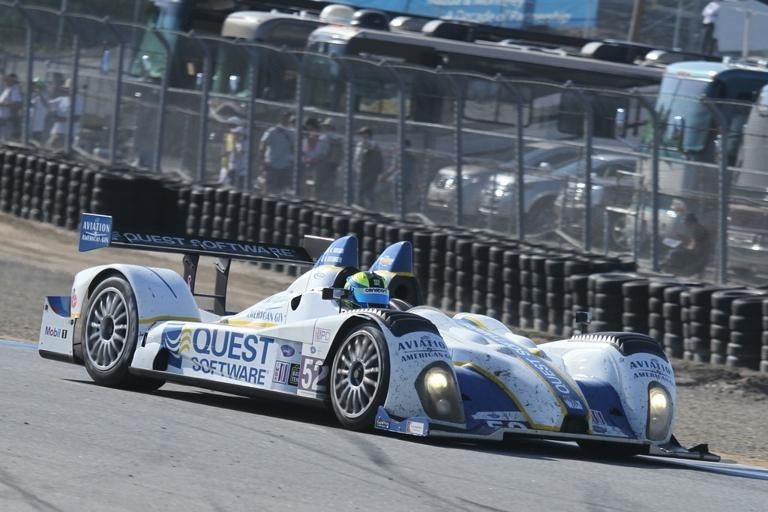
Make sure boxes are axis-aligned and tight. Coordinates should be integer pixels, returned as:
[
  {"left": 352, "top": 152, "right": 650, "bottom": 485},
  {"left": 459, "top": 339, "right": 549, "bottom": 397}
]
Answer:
[
  {"left": 0, "top": 70, "right": 84, "bottom": 152},
  {"left": 341, "top": 271, "right": 392, "bottom": 311},
  {"left": 218, "top": 108, "right": 417, "bottom": 215},
  {"left": 662, "top": 198, "right": 710, "bottom": 277}
]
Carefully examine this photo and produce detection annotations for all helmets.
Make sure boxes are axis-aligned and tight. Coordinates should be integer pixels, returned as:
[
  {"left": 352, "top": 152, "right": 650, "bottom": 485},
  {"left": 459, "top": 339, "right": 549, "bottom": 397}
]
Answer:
[{"left": 344, "top": 272, "right": 390, "bottom": 308}]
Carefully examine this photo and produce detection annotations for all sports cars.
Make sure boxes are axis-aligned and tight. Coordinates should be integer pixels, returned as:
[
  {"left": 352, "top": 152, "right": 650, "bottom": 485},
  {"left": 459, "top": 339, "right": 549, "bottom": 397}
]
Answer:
[{"left": 33, "top": 212, "right": 723, "bottom": 466}]
